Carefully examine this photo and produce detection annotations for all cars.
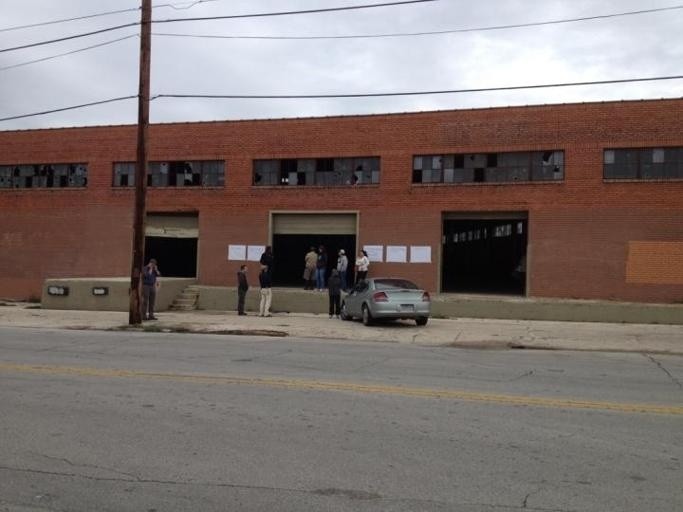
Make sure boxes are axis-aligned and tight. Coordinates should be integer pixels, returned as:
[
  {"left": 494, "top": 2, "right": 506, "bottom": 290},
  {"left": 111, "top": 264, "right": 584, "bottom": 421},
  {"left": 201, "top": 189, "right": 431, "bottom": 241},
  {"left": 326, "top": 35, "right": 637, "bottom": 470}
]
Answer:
[{"left": 339, "top": 276, "right": 431, "bottom": 326}]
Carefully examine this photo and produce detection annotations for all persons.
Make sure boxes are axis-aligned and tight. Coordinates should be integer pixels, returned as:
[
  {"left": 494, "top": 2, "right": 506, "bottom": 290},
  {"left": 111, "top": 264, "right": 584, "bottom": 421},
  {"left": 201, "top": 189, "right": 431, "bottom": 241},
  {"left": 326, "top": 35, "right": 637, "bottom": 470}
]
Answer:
[
  {"left": 314, "top": 244, "right": 327, "bottom": 292},
  {"left": 336, "top": 248, "right": 348, "bottom": 292},
  {"left": 140, "top": 259, "right": 161, "bottom": 321},
  {"left": 236, "top": 264, "right": 248, "bottom": 316},
  {"left": 259, "top": 245, "right": 273, "bottom": 287},
  {"left": 327, "top": 268, "right": 342, "bottom": 320},
  {"left": 257, "top": 265, "right": 273, "bottom": 317},
  {"left": 303, "top": 246, "right": 318, "bottom": 290},
  {"left": 354, "top": 249, "right": 370, "bottom": 284}
]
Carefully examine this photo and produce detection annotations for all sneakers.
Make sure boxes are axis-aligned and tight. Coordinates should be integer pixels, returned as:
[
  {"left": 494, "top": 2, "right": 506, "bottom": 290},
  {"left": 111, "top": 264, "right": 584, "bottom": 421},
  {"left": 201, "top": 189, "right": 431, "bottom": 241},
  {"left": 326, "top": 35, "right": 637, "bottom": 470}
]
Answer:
[
  {"left": 238, "top": 312, "right": 246, "bottom": 315},
  {"left": 148, "top": 316, "right": 158, "bottom": 320}
]
